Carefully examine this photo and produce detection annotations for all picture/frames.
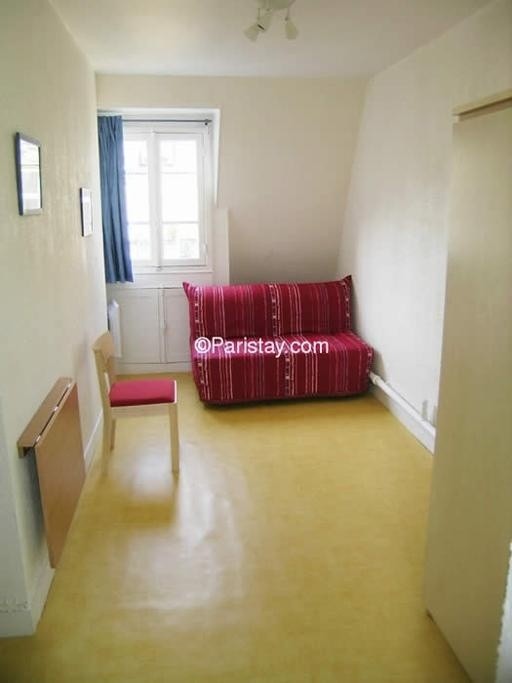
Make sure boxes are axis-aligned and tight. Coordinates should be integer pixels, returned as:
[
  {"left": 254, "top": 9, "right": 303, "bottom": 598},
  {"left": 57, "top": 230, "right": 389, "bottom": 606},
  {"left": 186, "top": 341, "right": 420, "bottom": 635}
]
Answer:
[{"left": 16, "top": 132, "right": 45, "bottom": 216}]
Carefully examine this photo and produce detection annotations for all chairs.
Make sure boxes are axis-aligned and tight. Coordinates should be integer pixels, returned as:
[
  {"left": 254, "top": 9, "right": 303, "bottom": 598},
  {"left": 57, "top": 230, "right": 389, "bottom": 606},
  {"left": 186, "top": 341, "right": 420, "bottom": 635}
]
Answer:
[{"left": 91, "top": 330, "right": 180, "bottom": 475}]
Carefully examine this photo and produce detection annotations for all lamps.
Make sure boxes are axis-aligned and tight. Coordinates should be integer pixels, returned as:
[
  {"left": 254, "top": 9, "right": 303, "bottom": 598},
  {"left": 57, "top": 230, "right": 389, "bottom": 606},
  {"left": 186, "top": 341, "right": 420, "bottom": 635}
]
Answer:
[{"left": 243, "top": 0, "right": 298, "bottom": 43}]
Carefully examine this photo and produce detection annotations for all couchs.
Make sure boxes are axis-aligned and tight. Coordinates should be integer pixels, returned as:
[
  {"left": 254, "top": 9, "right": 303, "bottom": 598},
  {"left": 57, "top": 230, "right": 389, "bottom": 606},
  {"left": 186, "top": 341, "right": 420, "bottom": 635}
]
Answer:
[{"left": 182, "top": 275, "right": 374, "bottom": 406}]
